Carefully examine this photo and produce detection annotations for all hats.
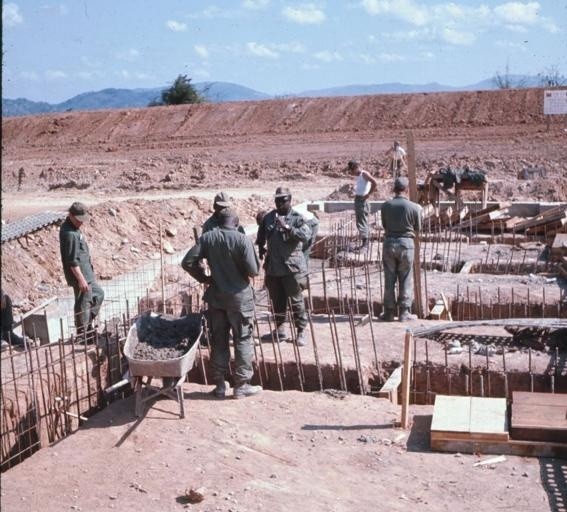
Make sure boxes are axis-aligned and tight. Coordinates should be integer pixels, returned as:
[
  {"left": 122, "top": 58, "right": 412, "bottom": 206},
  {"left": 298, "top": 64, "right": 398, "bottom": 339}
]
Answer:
[
  {"left": 69, "top": 203, "right": 89, "bottom": 223},
  {"left": 346, "top": 160, "right": 359, "bottom": 169},
  {"left": 216, "top": 190, "right": 232, "bottom": 206},
  {"left": 393, "top": 179, "right": 407, "bottom": 191},
  {"left": 272, "top": 187, "right": 291, "bottom": 199}
]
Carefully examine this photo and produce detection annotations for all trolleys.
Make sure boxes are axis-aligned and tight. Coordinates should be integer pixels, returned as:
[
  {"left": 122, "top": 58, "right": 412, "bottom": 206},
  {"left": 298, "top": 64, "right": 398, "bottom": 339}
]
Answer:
[{"left": 123, "top": 311, "right": 205, "bottom": 419}]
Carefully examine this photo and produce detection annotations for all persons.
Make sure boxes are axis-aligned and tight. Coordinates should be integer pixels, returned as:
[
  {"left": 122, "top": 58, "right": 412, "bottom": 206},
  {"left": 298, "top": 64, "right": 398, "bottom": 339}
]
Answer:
[
  {"left": 181, "top": 207, "right": 261, "bottom": 398},
  {"left": 255, "top": 186, "right": 312, "bottom": 344},
  {"left": 384, "top": 141, "right": 406, "bottom": 176},
  {"left": 378, "top": 178, "right": 424, "bottom": 322},
  {"left": 346, "top": 158, "right": 377, "bottom": 247},
  {"left": 200, "top": 191, "right": 247, "bottom": 235},
  {"left": 58, "top": 202, "right": 104, "bottom": 346},
  {"left": 253, "top": 207, "right": 320, "bottom": 282}
]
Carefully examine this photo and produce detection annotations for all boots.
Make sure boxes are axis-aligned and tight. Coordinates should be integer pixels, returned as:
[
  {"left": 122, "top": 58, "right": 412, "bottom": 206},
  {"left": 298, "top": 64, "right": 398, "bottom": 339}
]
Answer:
[
  {"left": 296, "top": 330, "right": 306, "bottom": 346},
  {"left": 264, "top": 324, "right": 287, "bottom": 342},
  {"left": 379, "top": 311, "right": 396, "bottom": 322},
  {"left": 233, "top": 379, "right": 262, "bottom": 399},
  {"left": 212, "top": 377, "right": 229, "bottom": 399},
  {"left": 399, "top": 308, "right": 417, "bottom": 322}
]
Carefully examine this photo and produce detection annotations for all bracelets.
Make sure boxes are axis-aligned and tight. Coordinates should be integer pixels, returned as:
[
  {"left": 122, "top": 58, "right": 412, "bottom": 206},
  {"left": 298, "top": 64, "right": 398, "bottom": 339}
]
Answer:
[{"left": 284, "top": 225, "right": 289, "bottom": 229}]
